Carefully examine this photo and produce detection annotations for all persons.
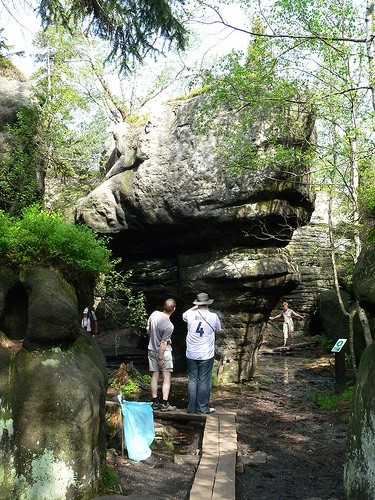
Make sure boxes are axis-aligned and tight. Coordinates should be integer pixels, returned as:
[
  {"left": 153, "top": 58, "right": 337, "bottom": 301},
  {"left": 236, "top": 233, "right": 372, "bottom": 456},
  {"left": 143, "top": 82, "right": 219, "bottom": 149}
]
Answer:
[
  {"left": 146, "top": 298, "right": 177, "bottom": 412},
  {"left": 82, "top": 308, "right": 98, "bottom": 339},
  {"left": 183, "top": 293, "right": 221, "bottom": 414},
  {"left": 269, "top": 302, "right": 303, "bottom": 346}
]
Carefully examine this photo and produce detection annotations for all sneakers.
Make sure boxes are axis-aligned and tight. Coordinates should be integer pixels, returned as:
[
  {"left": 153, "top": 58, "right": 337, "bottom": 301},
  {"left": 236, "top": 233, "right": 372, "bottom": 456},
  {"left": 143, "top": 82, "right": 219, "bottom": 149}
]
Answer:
[
  {"left": 152, "top": 401, "right": 177, "bottom": 412},
  {"left": 209, "top": 407, "right": 214, "bottom": 412}
]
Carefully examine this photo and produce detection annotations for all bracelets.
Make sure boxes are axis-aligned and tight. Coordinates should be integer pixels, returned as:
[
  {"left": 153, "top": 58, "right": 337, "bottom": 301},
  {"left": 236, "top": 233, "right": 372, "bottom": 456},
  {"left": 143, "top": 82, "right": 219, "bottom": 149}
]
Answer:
[{"left": 158, "top": 358, "right": 163, "bottom": 360}]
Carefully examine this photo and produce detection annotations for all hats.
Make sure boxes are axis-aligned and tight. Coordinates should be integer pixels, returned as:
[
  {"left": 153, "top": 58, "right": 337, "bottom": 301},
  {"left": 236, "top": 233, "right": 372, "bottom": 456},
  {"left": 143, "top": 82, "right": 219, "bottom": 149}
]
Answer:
[{"left": 192, "top": 292, "right": 215, "bottom": 306}]
